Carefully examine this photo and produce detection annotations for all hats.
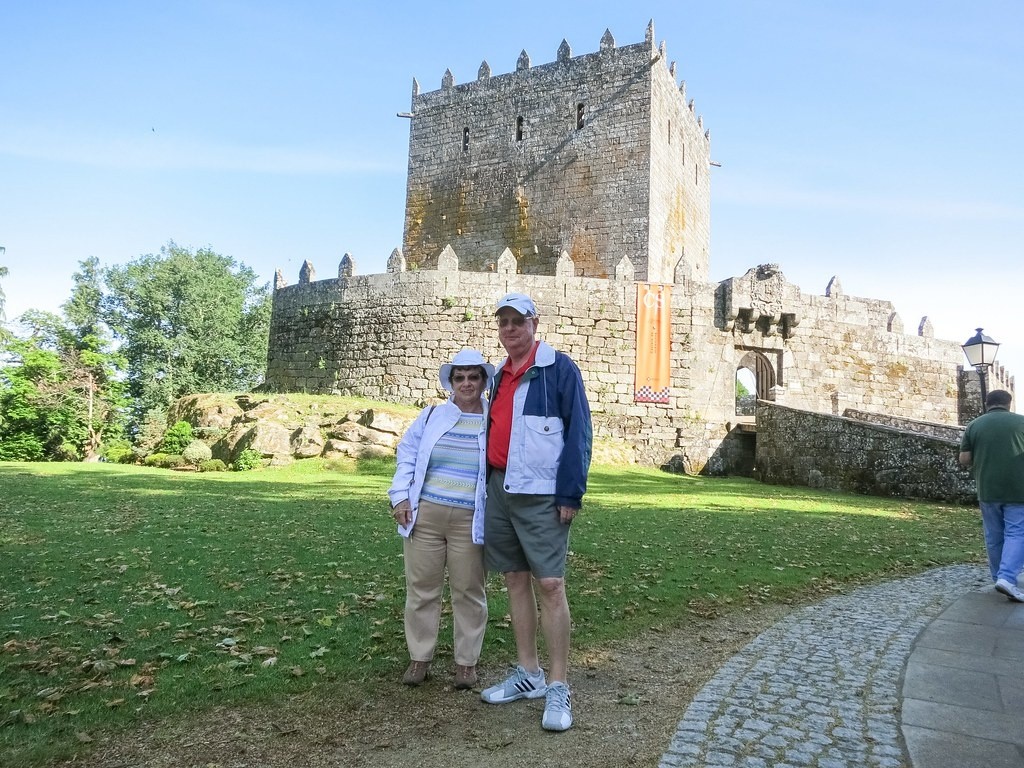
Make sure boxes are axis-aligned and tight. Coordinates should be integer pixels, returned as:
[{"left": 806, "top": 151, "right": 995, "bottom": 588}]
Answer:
[
  {"left": 439, "top": 350, "right": 495, "bottom": 392},
  {"left": 494, "top": 294, "right": 536, "bottom": 318}
]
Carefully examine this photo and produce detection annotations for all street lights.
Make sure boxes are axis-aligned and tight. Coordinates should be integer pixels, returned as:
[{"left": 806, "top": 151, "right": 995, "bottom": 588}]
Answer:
[{"left": 961, "top": 328, "right": 1000, "bottom": 414}]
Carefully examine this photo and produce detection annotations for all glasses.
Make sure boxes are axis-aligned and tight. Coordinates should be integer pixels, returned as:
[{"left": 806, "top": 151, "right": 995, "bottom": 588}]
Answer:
[
  {"left": 496, "top": 317, "right": 533, "bottom": 327},
  {"left": 449, "top": 373, "right": 483, "bottom": 382}
]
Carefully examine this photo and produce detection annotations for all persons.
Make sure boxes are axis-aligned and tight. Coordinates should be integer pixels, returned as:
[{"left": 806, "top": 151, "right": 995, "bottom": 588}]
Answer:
[
  {"left": 958, "top": 389, "right": 1024, "bottom": 603},
  {"left": 479, "top": 293, "right": 593, "bottom": 731},
  {"left": 389, "top": 350, "right": 495, "bottom": 687}
]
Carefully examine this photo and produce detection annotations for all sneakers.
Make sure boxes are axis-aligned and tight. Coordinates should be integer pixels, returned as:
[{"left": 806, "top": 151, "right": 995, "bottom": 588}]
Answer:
[
  {"left": 481, "top": 664, "right": 547, "bottom": 703},
  {"left": 542, "top": 681, "right": 572, "bottom": 730},
  {"left": 995, "top": 578, "right": 1024, "bottom": 602},
  {"left": 403, "top": 661, "right": 429, "bottom": 684},
  {"left": 454, "top": 664, "right": 476, "bottom": 688}
]
